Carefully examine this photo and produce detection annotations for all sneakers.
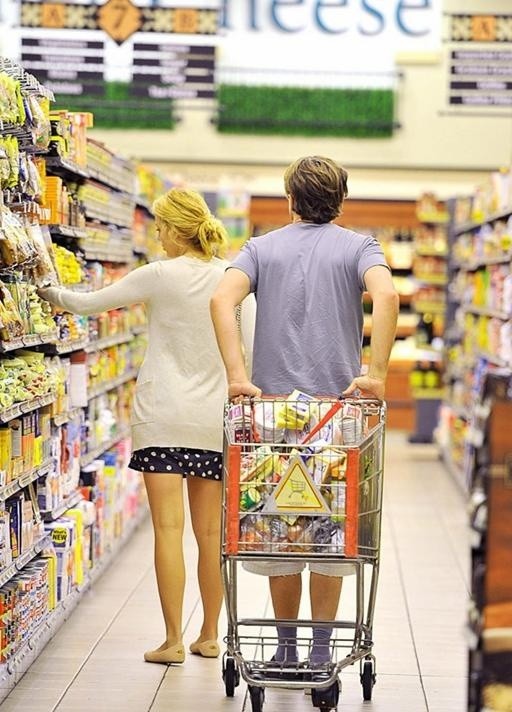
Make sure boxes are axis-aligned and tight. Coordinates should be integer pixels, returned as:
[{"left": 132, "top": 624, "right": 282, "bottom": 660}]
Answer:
[
  {"left": 264, "top": 656, "right": 305, "bottom": 681},
  {"left": 304, "top": 661, "right": 342, "bottom": 695}
]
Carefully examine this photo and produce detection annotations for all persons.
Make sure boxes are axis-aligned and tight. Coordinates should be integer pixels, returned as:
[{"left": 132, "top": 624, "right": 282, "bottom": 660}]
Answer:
[
  {"left": 35, "top": 185, "right": 257, "bottom": 667},
  {"left": 210, "top": 157, "right": 402, "bottom": 688}
]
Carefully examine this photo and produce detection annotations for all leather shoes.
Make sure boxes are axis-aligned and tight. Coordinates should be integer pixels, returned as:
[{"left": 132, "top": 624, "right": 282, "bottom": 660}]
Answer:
[
  {"left": 190, "top": 640, "right": 220, "bottom": 658},
  {"left": 144, "top": 645, "right": 185, "bottom": 663}
]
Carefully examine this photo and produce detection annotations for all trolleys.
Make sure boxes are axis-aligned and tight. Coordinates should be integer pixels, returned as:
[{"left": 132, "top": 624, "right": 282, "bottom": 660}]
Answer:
[{"left": 221, "top": 395, "right": 387, "bottom": 712}]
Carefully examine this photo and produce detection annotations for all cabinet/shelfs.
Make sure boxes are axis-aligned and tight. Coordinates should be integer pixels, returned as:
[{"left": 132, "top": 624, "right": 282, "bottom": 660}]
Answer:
[{"left": 249, "top": 191, "right": 452, "bottom": 433}]
